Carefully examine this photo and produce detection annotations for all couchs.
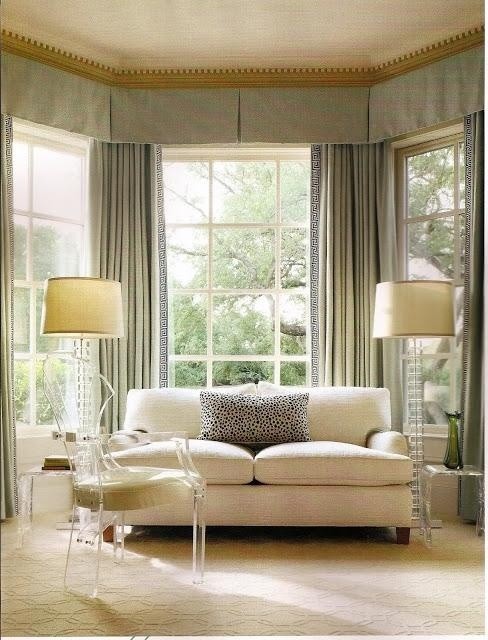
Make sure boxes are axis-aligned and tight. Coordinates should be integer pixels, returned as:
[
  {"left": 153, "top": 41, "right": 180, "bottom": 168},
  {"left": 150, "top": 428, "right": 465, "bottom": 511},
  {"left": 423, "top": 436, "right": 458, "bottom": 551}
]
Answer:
[{"left": 96, "top": 387, "right": 413, "bottom": 543}]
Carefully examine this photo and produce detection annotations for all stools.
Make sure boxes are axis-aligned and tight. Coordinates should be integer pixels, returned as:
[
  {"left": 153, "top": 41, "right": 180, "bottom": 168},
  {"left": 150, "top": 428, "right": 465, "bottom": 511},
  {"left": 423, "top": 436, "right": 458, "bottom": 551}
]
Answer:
[
  {"left": 418, "top": 464, "right": 485, "bottom": 542},
  {"left": 15, "top": 468, "right": 91, "bottom": 547}
]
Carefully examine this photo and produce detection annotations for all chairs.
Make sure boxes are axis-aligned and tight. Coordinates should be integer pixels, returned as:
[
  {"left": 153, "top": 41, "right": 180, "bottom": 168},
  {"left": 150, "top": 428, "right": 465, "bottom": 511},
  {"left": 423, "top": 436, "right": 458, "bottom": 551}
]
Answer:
[{"left": 38, "top": 351, "right": 207, "bottom": 604}]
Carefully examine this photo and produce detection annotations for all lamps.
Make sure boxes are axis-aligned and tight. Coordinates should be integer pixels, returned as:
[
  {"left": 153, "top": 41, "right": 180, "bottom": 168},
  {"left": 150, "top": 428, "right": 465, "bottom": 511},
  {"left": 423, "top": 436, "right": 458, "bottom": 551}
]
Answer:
[
  {"left": 372, "top": 282, "right": 454, "bottom": 546},
  {"left": 43, "top": 275, "right": 127, "bottom": 540}
]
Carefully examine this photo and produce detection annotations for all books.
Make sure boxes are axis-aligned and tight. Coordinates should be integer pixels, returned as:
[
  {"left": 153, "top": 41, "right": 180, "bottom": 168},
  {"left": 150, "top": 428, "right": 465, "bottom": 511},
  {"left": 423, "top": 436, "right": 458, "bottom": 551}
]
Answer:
[
  {"left": 43, "top": 463, "right": 71, "bottom": 467},
  {"left": 44, "top": 461, "right": 70, "bottom": 463},
  {"left": 42, "top": 466, "right": 72, "bottom": 471},
  {"left": 44, "top": 454, "right": 70, "bottom": 460}
]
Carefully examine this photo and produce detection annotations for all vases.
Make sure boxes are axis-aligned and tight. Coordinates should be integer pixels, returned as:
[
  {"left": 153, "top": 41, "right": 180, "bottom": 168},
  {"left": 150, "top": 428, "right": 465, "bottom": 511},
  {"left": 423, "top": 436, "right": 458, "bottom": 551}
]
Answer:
[{"left": 439, "top": 405, "right": 462, "bottom": 468}]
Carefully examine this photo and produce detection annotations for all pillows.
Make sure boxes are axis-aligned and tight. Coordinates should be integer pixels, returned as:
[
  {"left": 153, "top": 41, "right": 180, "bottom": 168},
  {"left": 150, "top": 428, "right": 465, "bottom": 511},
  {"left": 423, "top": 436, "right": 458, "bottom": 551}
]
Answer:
[{"left": 199, "top": 391, "right": 311, "bottom": 445}]
